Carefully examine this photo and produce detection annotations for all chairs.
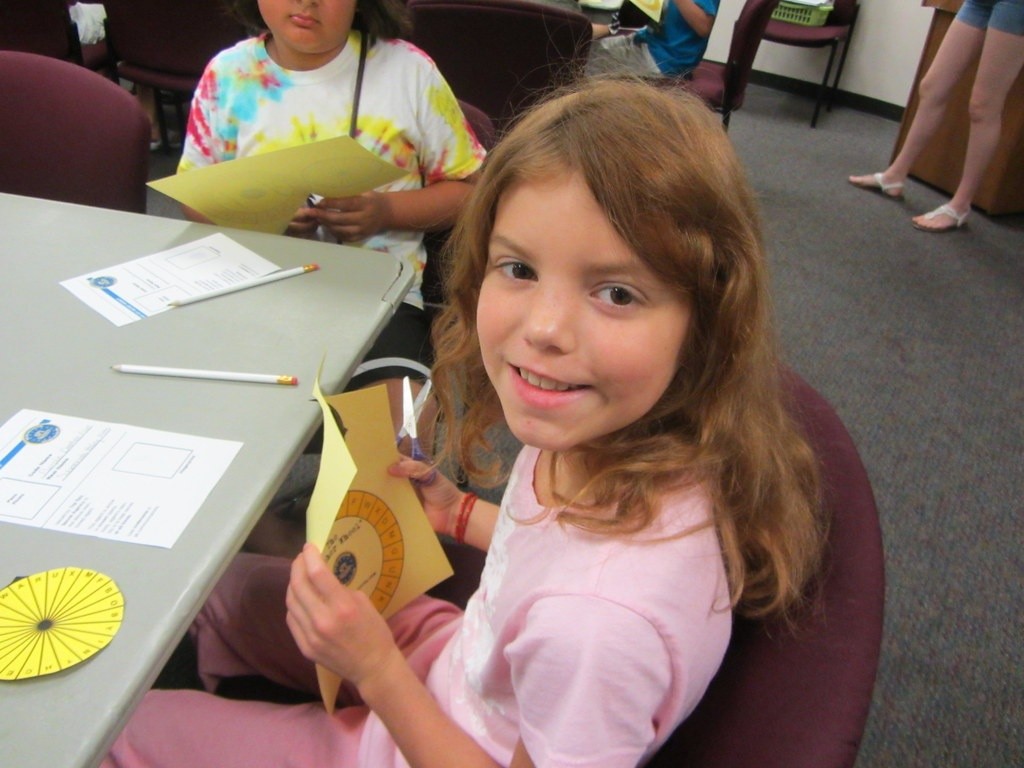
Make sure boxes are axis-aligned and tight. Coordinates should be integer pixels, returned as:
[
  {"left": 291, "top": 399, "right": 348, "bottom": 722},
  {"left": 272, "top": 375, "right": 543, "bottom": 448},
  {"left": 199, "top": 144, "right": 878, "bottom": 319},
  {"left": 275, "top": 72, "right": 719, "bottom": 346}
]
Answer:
[
  {"left": 0, "top": 50, "right": 151, "bottom": 214},
  {"left": 403, "top": 1, "right": 594, "bottom": 148},
  {"left": 728, "top": 0, "right": 859, "bottom": 129},
  {"left": 653, "top": 346, "right": 885, "bottom": 768},
  {"left": 0, "top": 1, "right": 122, "bottom": 88},
  {"left": 648, "top": 1, "right": 778, "bottom": 136},
  {"left": 117, "top": 0, "right": 248, "bottom": 156}
]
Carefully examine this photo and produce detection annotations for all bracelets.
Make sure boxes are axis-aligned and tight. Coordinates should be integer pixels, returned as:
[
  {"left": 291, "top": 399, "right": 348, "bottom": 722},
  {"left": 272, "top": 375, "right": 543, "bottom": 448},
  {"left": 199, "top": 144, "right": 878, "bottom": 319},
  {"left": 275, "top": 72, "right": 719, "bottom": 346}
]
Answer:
[{"left": 454, "top": 492, "right": 480, "bottom": 545}]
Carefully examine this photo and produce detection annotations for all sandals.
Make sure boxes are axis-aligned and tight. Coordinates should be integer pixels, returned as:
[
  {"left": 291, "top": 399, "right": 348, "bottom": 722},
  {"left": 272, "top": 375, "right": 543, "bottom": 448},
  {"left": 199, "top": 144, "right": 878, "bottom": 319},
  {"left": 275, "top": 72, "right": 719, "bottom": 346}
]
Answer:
[
  {"left": 913, "top": 203, "right": 971, "bottom": 232},
  {"left": 848, "top": 174, "right": 907, "bottom": 201}
]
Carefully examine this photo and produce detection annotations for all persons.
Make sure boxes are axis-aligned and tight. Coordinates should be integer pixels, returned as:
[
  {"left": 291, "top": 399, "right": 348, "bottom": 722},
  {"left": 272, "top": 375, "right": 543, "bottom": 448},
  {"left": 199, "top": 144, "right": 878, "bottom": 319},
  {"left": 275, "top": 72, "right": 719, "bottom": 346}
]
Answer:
[
  {"left": 101, "top": 79, "right": 832, "bottom": 767},
  {"left": 171, "top": 0, "right": 489, "bottom": 560},
  {"left": 847, "top": 0, "right": 1024, "bottom": 234},
  {"left": 584, "top": 0, "right": 721, "bottom": 82}
]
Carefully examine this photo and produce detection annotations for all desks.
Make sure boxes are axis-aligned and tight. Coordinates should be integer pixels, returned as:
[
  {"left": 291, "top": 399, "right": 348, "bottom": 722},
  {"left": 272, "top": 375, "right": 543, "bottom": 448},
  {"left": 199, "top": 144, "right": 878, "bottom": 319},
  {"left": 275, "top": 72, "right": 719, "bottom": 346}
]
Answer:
[{"left": 0, "top": 187, "right": 417, "bottom": 767}]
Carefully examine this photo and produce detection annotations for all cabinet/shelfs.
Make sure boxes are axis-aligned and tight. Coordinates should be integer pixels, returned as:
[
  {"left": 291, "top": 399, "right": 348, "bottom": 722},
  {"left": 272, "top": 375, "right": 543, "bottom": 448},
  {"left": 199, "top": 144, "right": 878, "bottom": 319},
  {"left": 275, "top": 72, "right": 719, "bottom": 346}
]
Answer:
[{"left": 885, "top": 1, "right": 1024, "bottom": 218}]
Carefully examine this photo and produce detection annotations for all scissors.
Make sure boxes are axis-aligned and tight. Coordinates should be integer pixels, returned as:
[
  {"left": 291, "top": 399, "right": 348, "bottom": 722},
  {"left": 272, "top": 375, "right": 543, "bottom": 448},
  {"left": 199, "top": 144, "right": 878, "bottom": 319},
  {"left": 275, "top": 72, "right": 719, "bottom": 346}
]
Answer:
[{"left": 395, "top": 377, "right": 438, "bottom": 484}]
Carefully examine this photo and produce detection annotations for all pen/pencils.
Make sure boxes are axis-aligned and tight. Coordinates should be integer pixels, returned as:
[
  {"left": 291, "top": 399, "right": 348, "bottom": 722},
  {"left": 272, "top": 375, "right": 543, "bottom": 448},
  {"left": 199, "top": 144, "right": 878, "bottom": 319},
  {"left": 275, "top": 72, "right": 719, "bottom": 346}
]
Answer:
[
  {"left": 167, "top": 264, "right": 319, "bottom": 306},
  {"left": 110, "top": 364, "right": 298, "bottom": 386}
]
